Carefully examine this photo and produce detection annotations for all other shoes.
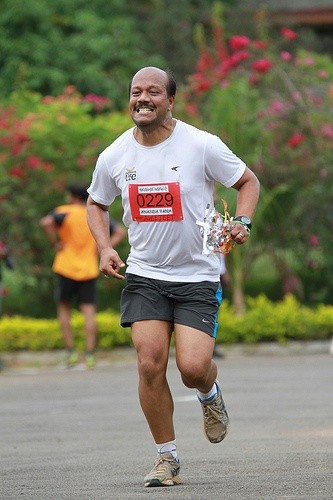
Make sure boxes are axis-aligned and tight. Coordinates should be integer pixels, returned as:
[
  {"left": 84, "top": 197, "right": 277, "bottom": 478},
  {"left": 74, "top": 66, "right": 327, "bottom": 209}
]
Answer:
[
  {"left": 62, "top": 350, "right": 78, "bottom": 366},
  {"left": 85, "top": 355, "right": 94, "bottom": 369}
]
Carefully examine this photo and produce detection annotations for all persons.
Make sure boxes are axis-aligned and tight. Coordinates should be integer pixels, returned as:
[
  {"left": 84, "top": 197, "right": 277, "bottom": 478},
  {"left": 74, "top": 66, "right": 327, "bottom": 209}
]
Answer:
[
  {"left": 41, "top": 182, "right": 127, "bottom": 371},
  {"left": 86, "top": 67, "right": 260, "bottom": 487}
]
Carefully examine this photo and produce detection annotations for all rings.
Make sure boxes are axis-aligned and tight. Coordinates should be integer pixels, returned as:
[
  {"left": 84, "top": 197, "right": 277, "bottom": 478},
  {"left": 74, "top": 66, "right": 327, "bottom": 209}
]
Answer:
[
  {"left": 239, "top": 233, "right": 244, "bottom": 237},
  {"left": 105, "top": 274, "right": 111, "bottom": 279}
]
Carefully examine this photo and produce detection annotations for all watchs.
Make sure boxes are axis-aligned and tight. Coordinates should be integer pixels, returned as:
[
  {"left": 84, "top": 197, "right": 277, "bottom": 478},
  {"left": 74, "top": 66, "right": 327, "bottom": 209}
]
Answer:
[{"left": 232, "top": 216, "right": 252, "bottom": 230}]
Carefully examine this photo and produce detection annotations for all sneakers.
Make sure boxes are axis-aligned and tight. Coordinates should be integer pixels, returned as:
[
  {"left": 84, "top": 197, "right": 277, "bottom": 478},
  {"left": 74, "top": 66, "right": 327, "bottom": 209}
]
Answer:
[
  {"left": 143, "top": 451, "right": 184, "bottom": 487},
  {"left": 196, "top": 379, "right": 228, "bottom": 443}
]
